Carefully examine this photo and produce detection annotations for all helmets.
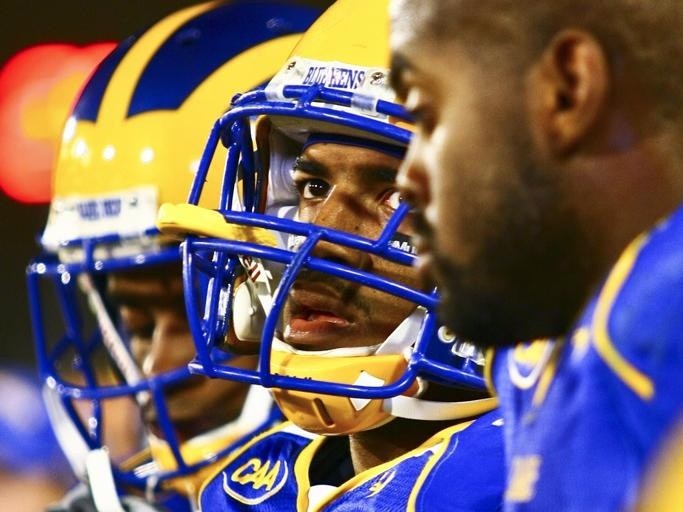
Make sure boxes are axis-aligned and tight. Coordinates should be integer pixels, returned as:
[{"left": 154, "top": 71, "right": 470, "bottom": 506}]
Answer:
[
  {"left": 25, "top": 2, "right": 321, "bottom": 512},
  {"left": 157, "top": 0, "right": 497, "bottom": 436}
]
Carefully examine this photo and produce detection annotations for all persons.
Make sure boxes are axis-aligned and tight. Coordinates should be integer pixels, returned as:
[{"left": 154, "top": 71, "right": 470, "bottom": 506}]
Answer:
[
  {"left": 383, "top": 0, "right": 683, "bottom": 510},
  {"left": 24, "top": 0, "right": 331, "bottom": 511},
  {"left": 192, "top": 1, "right": 494, "bottom": 512}
]
